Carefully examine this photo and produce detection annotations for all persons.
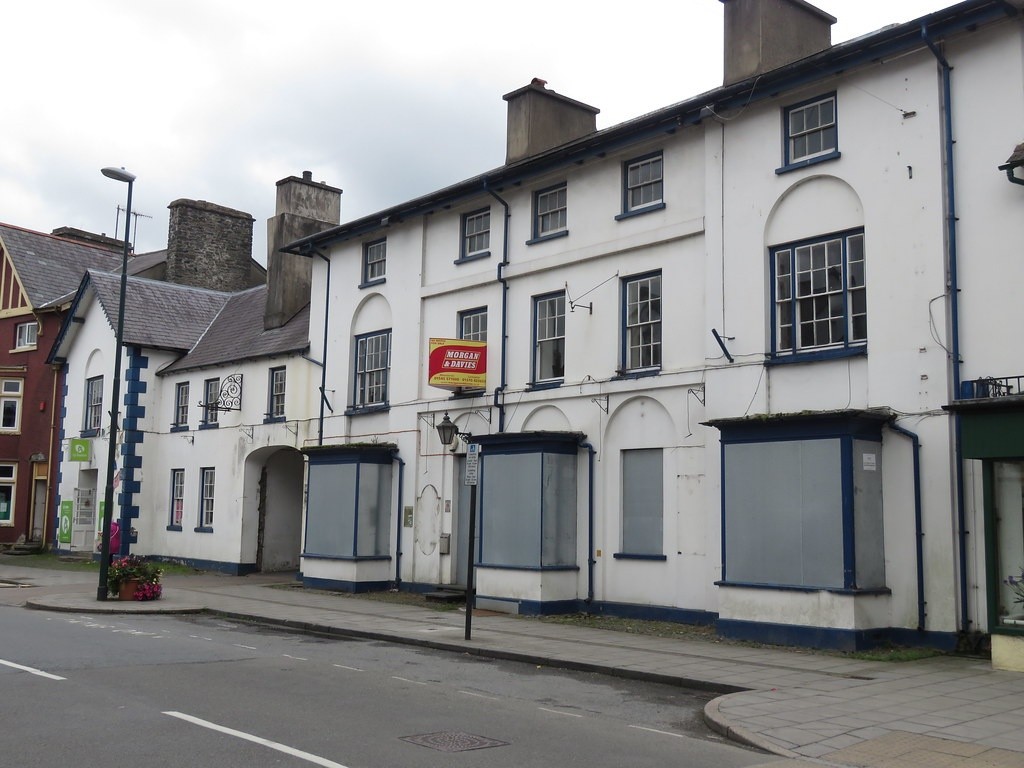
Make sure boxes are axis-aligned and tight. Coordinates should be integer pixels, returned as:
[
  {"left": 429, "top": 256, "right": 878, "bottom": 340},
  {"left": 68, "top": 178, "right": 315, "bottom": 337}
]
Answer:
[{"left": 98, "top": 522, "right": 120, "bottom": 565}]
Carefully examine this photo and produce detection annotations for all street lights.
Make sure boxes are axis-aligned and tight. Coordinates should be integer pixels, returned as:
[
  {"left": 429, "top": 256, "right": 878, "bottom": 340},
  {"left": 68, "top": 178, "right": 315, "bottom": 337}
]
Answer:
[
  {"left": 433, "top": 409, "right": 479, "bottom": 642},
  {"left": 96, "top": 165, "right": 137, "bottom": 602}
]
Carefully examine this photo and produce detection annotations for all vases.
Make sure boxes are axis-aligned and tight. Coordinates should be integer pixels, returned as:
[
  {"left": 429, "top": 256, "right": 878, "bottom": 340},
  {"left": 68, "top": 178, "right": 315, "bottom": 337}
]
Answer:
[{"left": 119, "top": 577, "right": 142, "bottom": 601}]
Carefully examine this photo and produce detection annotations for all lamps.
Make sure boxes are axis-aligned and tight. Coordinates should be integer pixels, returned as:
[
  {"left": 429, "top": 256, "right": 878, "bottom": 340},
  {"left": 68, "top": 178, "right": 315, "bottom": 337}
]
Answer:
[
  {"left": 436, "top": 410, "right": 472, "bottom": 445},
  {"left": 381, "top": 216, "right": 394, "bottom": 228}
]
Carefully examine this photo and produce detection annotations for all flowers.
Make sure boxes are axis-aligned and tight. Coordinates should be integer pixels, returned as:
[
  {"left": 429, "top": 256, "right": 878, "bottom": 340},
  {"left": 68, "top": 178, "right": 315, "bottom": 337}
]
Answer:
[{"left": 106, "top": 557, "right": 165, "bottom": 601}]
